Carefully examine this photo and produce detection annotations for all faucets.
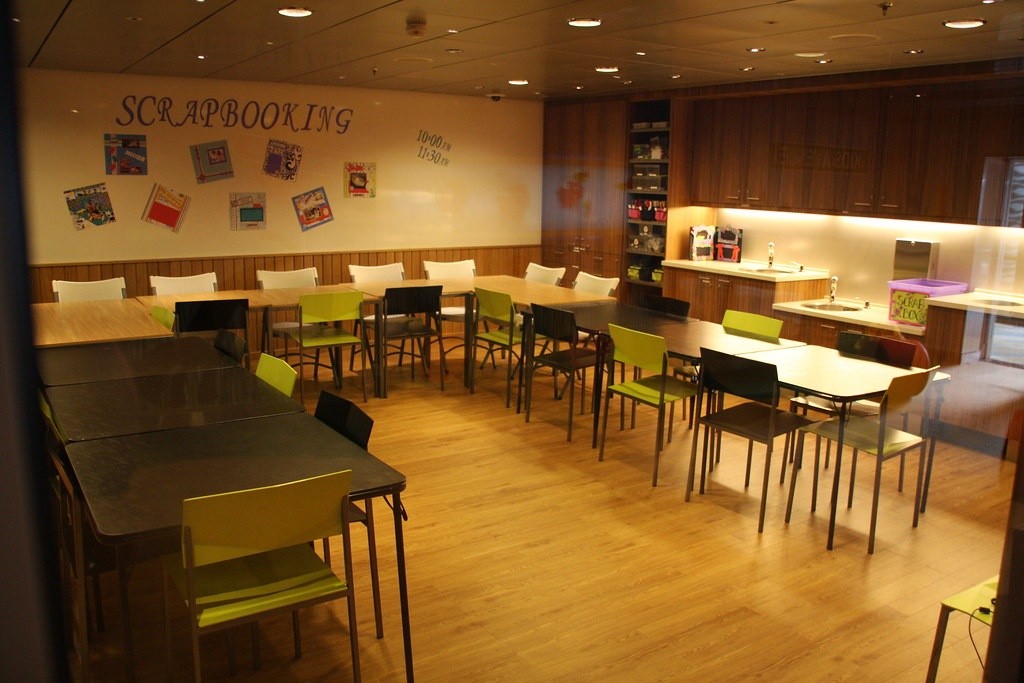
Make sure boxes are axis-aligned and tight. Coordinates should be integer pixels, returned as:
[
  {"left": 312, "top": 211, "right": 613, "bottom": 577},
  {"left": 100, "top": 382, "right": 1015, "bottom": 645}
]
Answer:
[
  {"left": 768, "top": 242, "right": 774, "bottom": 267},
  {"left": 829, "top": 276, "right": 838, "bottom": 302}
]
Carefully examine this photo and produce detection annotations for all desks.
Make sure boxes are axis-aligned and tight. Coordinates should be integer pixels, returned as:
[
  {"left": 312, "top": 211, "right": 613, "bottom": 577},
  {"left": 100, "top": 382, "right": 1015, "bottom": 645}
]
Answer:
[
  {"left": 44, "top": 367, "right": 307, "bottom": 639},
  {"left": 590, "top": 320, "right": 807, "bottom": 501},
  {"left": 65, "top": 414, "right": 414, "bottom": 683},
  {"left": 33, "top": 298, "right": 174, "bottom": 347},
  {"left": 134, "top": 289, "right": 270, "bottom": 380},
  {"left": 255, "top": 284, "right": 383, "bottom": 399},
  {"left": 733, "top": 345, "right": 951, "bottom": 551},
  {"left": 480, "top": 283, "right": 616, "bottom": 404},
  {"left": 340, "top": 277, "right": 475, "bottom": 397},
  {"left": 522, "top": 298, "right": 699, "bottom": 449},
  {"left": 37, "top": 335, "right": 240, "bottom": 387},
  {"left": 435, "top": 272, "right": 535, "bottom": 390}
]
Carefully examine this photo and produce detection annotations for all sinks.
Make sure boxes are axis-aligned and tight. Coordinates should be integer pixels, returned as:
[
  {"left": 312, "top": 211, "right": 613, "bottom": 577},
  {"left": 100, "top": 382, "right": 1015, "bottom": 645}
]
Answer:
[
  {"left": 800, "top": 303, "right": 863, "bottom": 311},
  {"left": 738, "top": 268, "right": 795, "bottom": 274}
]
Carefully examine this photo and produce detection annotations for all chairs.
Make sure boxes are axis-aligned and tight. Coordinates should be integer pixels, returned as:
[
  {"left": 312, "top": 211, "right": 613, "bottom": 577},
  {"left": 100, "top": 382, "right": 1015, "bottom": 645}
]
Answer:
[
  {"left": 32, "top": 259, "right": 940, "bottom": 682},
  {"left": 927, "top": 575, "right": 999, "bottom": 683}
]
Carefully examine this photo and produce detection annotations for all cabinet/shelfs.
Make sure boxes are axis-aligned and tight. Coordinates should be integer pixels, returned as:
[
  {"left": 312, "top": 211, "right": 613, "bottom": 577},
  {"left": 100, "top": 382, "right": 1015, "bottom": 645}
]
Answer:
[{"left": 542, "top": 52, "right": 1024, "bottom": 462}]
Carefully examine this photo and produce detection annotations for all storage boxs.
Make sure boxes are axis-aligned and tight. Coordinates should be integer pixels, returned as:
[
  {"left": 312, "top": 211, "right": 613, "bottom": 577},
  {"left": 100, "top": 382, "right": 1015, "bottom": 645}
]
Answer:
[{"left": 888, "top": 277, "right": 968, "bottom": 325}]
